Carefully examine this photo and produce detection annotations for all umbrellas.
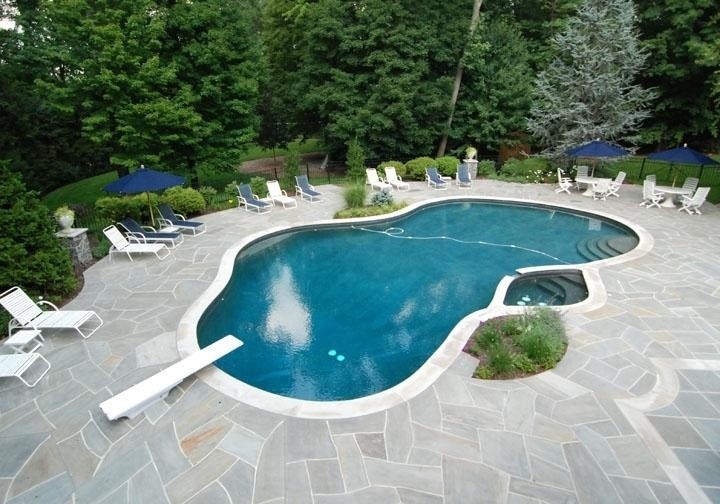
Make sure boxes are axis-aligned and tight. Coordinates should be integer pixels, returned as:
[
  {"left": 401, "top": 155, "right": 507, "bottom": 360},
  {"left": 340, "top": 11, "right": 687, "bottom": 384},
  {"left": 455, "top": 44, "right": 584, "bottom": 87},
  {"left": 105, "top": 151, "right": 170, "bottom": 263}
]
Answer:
[
  {"left": 647, "top": 143, "right": 720, "bottom": 188},
  {"left": 564, "top": 138, "right": 630, "bottom": 176},
  {"left": 102, "top": 165, "right": 186, "bottom": 227}
]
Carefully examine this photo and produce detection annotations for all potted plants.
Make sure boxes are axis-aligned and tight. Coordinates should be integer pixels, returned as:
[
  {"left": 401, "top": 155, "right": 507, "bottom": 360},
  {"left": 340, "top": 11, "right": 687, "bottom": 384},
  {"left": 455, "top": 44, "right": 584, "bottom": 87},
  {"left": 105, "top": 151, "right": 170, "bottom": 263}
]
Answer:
[
  {"left": 464, "top": 145, "right": 478, "bottom": 163},
  {"left": 51, "top": 204, "right": 79, "bottom": 236}
]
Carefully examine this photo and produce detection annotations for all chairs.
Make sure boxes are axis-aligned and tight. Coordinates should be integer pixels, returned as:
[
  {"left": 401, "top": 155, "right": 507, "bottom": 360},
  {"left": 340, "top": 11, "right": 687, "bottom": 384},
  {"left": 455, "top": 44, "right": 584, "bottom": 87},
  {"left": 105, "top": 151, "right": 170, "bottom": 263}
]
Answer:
[
  {"left": 364, "top": 166, "right": 395, "bottom": 195},
  {"left": 554, "top": 164, "right": 714, "bottom": 216},
  {"left": 424, "top": 165, "right": 449, "bottom": 191},
  {"left": 265, "top": 178, "right": 299, "bottom": 211},
  {"left": 0, "top": 351, "right": 53, "bottom": 388},
  {"left": 0, "top": 284, "right": 105, "bottom": 341},
  {"left": 116, "top": 215, "right": 185, "bottom": 250},
  {"left": 102, "top": 224, "right": 172, "bottom": 263},
  {"left": 234, "top": 182, "right": 273, "bottom": 216},
  {"left": 153, "top": 201, "right": 207, "bottom": 238},
  {"left": 293, "top": 174, "right": 325, "bottom": 205},
  {"left": 385, "top": 165, "right": 411, "bottom": 193},
  {"left": 455, "top": 162, "right": 474, "bottom": 191}
]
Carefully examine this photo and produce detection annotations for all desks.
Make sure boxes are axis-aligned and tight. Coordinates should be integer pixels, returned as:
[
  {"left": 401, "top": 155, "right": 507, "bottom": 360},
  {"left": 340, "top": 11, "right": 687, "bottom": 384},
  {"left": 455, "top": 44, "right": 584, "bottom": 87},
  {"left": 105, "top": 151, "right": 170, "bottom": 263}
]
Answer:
[
  {"left": 442, "top": 176, "right": 453, "bottom": 187},
  {"left": 260, "top": 197, "right": 276, "bottom": 207},
  {"left": 1, "top": 329, "right": 46, "bottom": 355}
]
